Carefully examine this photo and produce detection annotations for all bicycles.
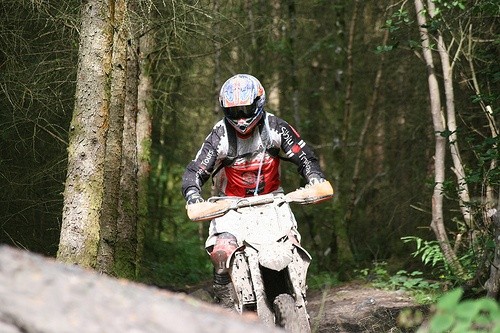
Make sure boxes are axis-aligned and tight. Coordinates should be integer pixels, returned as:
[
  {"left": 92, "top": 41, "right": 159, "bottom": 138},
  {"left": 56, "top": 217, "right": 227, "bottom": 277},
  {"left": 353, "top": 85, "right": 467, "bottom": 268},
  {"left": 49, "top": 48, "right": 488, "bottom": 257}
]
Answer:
[{"left": 187, "top": 181, "right": 334, "bottom": 333}]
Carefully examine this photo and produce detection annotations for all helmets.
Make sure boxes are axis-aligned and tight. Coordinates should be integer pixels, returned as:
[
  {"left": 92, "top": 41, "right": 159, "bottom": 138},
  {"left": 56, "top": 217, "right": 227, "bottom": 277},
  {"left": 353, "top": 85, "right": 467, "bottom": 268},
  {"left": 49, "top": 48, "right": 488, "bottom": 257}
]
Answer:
[{"left": 219, "top": 74, "right": 265, "bottom": 135}]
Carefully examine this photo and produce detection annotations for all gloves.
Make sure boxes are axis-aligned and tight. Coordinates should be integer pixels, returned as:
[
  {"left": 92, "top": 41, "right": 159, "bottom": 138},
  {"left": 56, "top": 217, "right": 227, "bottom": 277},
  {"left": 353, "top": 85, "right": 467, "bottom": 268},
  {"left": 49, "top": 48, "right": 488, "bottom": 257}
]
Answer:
[
  {"left": 309, "top": 173, "right": 326, "bottom": 186},
  {"left": 185, "top": 186, "right": 204, "bottom": 204}
]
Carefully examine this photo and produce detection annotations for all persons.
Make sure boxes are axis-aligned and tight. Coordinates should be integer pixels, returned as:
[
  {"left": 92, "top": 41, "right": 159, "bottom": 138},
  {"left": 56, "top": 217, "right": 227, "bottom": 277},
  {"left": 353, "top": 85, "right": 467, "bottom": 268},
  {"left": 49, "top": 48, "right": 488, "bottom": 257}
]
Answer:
[{"left": 181, "top": 74, "right": 326, "bottom": 320}]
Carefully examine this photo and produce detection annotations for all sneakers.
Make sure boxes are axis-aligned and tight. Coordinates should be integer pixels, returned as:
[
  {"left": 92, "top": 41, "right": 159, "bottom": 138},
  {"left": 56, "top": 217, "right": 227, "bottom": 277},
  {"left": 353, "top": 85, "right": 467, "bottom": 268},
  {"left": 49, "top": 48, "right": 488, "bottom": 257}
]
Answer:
[{"left": 213, "top": 267, "right": 232, "bottom": 287}]
